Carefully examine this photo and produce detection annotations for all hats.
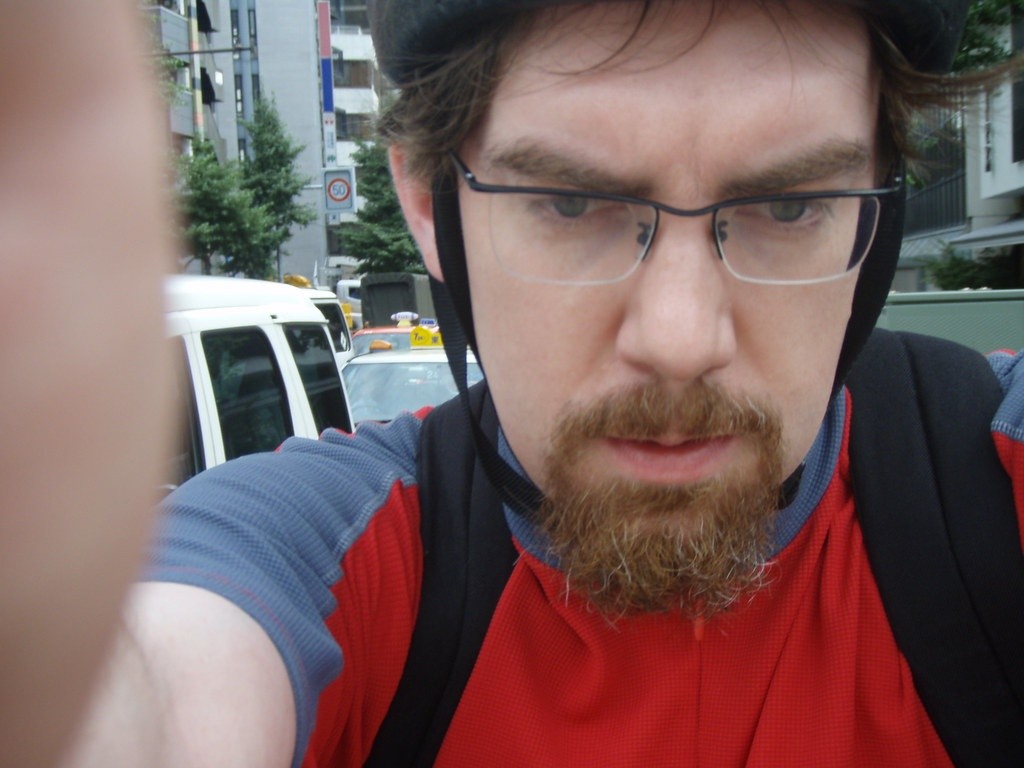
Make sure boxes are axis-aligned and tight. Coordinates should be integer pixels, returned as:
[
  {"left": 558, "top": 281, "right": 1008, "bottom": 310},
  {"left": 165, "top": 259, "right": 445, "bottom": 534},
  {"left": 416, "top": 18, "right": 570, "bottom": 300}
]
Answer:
[{"left": 366, "top": 0, "right": 969, "bottom": 90}]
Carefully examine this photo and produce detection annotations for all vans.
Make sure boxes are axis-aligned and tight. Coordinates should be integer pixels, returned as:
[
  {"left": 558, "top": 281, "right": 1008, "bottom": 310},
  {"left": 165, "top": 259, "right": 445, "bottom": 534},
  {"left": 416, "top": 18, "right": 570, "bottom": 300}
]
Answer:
[
  {"left": 166, "top": 275, "right": 354, "bottom": 499},
  {"left": 300, "top": 285, "right": 353, "bottom": 372}
]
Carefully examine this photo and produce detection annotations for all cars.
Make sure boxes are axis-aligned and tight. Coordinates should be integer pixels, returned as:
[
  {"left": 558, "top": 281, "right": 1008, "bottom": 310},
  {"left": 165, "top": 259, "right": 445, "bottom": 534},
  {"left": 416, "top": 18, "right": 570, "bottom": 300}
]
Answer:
[{"left": 343, "top": 322, "right": 487, "bottom": 433}]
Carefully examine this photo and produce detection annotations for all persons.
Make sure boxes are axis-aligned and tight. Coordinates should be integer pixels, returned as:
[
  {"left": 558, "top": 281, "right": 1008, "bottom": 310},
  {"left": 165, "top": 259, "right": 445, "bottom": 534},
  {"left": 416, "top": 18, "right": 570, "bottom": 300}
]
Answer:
[{"left": 0, "top": 0, "right": 1024, "bottom": 768}]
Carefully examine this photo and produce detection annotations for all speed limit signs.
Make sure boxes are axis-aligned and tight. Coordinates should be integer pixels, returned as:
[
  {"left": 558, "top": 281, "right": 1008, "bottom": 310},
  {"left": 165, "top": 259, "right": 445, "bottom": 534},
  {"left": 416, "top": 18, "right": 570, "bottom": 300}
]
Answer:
[{"left": 328, "top": 178, "right": 350, "bottom": 201}]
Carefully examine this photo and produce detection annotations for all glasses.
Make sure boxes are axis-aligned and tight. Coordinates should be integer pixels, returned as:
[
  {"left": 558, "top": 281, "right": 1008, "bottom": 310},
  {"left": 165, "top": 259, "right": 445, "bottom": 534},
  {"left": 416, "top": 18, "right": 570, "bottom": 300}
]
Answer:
[{"left": 449, "top": 147, "right": 898, "bottom": 287}]
